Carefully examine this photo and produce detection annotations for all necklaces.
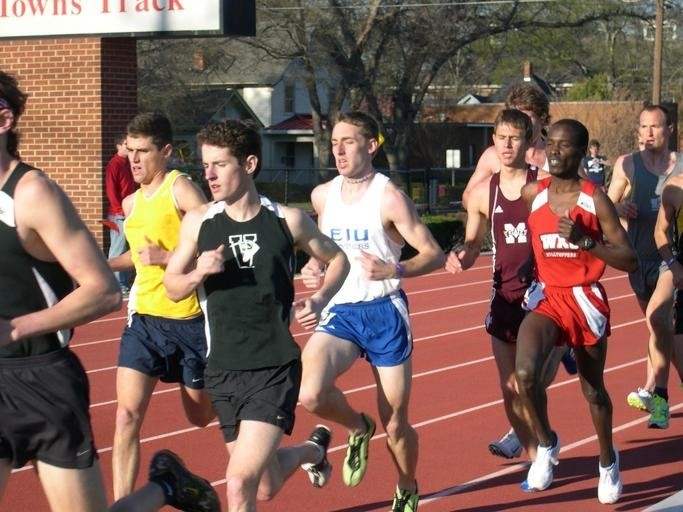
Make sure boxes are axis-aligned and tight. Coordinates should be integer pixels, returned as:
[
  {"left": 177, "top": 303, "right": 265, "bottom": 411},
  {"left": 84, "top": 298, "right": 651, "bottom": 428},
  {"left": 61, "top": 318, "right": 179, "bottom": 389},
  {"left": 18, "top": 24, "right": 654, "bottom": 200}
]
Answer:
[{"left": 344, "top": 167, "right": 375, "bottom": 184}]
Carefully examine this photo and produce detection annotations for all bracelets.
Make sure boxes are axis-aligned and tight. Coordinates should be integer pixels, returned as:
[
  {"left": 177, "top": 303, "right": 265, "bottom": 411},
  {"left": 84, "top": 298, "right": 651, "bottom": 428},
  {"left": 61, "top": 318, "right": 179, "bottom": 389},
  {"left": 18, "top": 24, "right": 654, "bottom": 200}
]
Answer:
[{"left": 388, "top": 262, "right": 407, "bottom": 278}]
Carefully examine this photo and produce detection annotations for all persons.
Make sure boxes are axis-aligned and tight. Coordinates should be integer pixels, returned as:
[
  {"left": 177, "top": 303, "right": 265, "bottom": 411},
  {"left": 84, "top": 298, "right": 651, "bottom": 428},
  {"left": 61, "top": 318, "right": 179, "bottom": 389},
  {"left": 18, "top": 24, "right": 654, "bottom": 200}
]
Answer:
[
  {"left": 299, "top": 112, "right": 445, "bottom": 511},
  {"left": 105, "top": 133, "right": 141, "bottom": 301},
  {"left": 608, "top": 104, "right": 682, "bottom": 430},
  {"left": 160, "top": 117, "right": 351, "bottom": 512},
  {"left": 106, "top": 108, "right": 217, "bottom": 499},
  {"left": 444, "top": 83, "right": 623, "bottom": 504},
  {"left": 0, "top": 70, "right": 222, "bottom": 511}
]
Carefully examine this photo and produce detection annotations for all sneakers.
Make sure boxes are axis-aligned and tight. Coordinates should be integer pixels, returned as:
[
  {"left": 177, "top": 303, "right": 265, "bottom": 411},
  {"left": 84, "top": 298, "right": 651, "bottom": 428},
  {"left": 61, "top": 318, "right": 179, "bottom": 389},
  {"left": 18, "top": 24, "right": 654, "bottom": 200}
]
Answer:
[
  {"left": 489, "top": 424, "right": 524, "bottom": 460},
  {"left": 299, "top": 425, "right": 333, "bottom": 489},
  {"left": 527, "top": 430, "right": 562, "bottom": 492},
  {"left": 342, "top": 412, "right": 377, "bottom": 490},
  {"left": 646, "top": 395, "right": 673, "bottom": 429},
  {"left": 626, "top": 386, "right": 654, "bottom": 415},
  {"left": 596, "top": 448, "right": 624, "bottom": 507},
  {"left": 150, "top": 448, "right": 221, "bottom": 512},
  {"left": 388, "top": 476, "right": 421, "bottom": 511}
]
